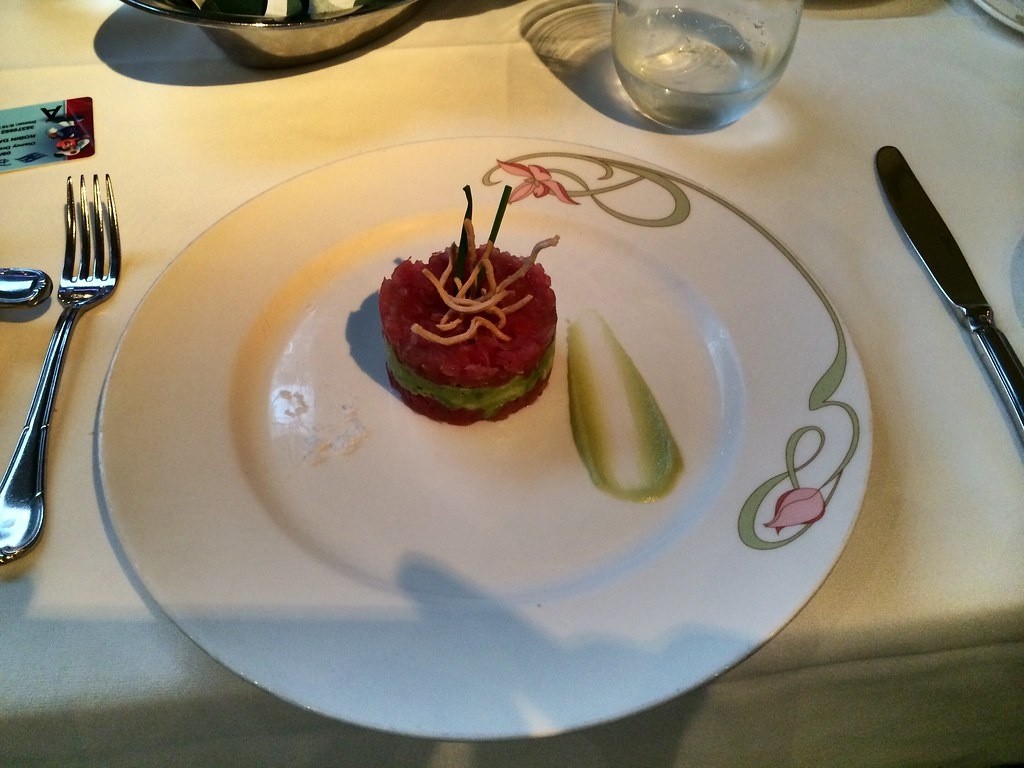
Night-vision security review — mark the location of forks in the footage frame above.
[0,176,122,566]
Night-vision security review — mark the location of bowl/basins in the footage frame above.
[121,0,421,69]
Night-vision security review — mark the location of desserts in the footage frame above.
[380,183,562,427]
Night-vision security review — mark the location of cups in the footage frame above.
[612,1,803,133]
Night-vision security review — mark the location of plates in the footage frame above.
[95,136,872,739]
[973,0,1024,34]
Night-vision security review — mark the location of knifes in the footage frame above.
[874,146,1024,448]
